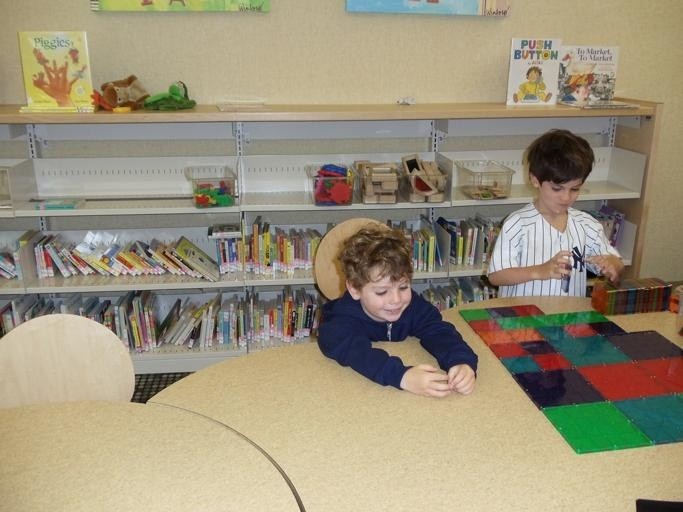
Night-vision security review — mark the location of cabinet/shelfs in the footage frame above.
[1,105,249,375]
[242,98,665,356]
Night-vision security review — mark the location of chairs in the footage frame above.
[0,314,136,402]
[312,218,395,300]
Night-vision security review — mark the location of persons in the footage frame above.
[512,67,552,102]
[316,228,479,399]
[485,130,626,297]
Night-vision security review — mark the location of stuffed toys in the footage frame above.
[194,181,234,209]
[91,75,197,112]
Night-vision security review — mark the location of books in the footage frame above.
[556,46,620,104]
[557,99,640,110]
[581,205,626,249]
[207,215,323,275]
[16,30,96,115]
[0,229,219,282]
[19,198,85,210]
[506,37,562,107]
[0,285,327,353]
[386,213,502,314]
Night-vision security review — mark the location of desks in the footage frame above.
[144,296,682,510]
[0,402,303,511]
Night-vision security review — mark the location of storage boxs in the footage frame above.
[451,157,516,203]
[303,162,356,208]
[181,163,238,209]
[356,158,403,207]
[394,160,449,205]
